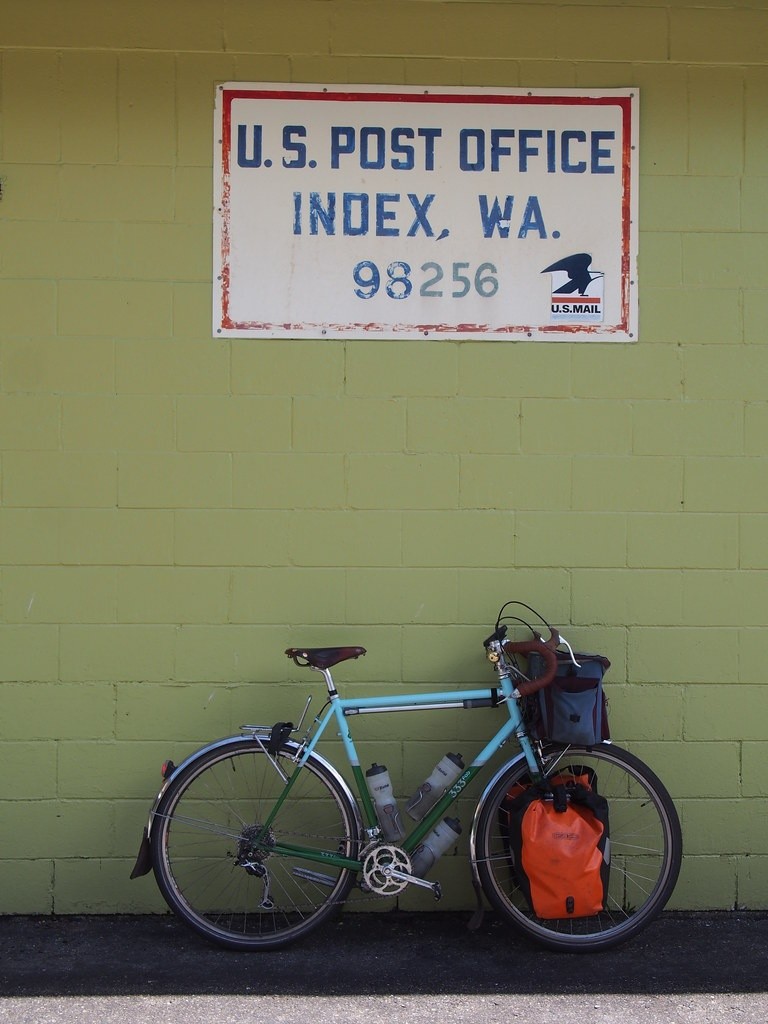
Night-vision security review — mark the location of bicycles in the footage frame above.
[127,602,684,956]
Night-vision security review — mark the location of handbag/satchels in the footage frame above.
[497,765,610,920]
[526,649,610,746]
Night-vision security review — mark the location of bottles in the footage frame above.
[407,752,470,821]
[391,817,461,883]
[365,761,406,842]
